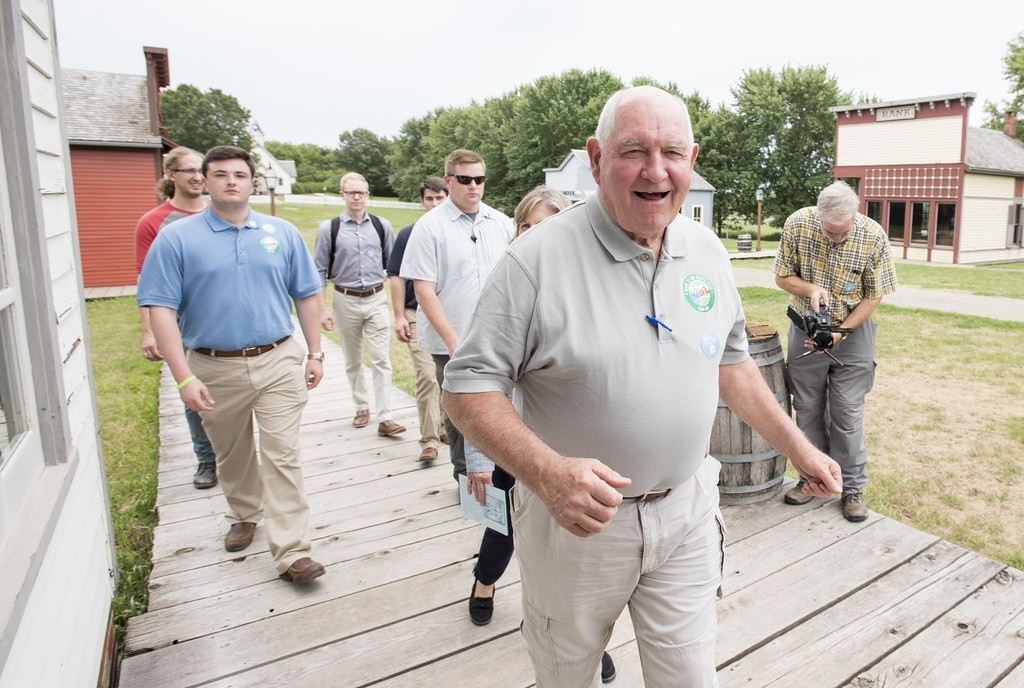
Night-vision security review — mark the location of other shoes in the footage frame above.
[469,578,496,626]
[601,651,616,684]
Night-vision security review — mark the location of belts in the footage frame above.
[194,336,291,360]
[334,284,384,298]
[622,489,670,504]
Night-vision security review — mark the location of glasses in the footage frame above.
[820,218,855,238]
[172,169,204,178]
[446,172,486,185]
[343,191,369,198]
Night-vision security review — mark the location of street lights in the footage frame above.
[755,189,764,251]
[264,163,277,215]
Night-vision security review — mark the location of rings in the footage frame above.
[144,353,148,357]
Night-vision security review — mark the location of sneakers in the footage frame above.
[842,493,868,521]
[784,478,815,505]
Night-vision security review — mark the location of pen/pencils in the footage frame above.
[646,315,673,333]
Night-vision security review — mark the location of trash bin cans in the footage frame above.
[711,321,791,505]
[737,234,753,253]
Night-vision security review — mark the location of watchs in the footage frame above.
[307,352,324,362]
[837,327,847,341]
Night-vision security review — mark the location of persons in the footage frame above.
[137,145,325,583]
[464,189,616,682]
[135,146,219,489]
[388,176,450,462]
[398,150,518,505]
[439,86,842,688]
[313,172,406,435]
[772,180,896,522]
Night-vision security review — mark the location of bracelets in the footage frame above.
[177,375,196,391]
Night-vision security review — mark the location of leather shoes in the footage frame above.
[353,409,369,427]
[419,447,438,462]
[378,420,406,436]
[225,521,257,552]
[440,434,450,445]
[279,558,326,585]
[194,462,217,488]
